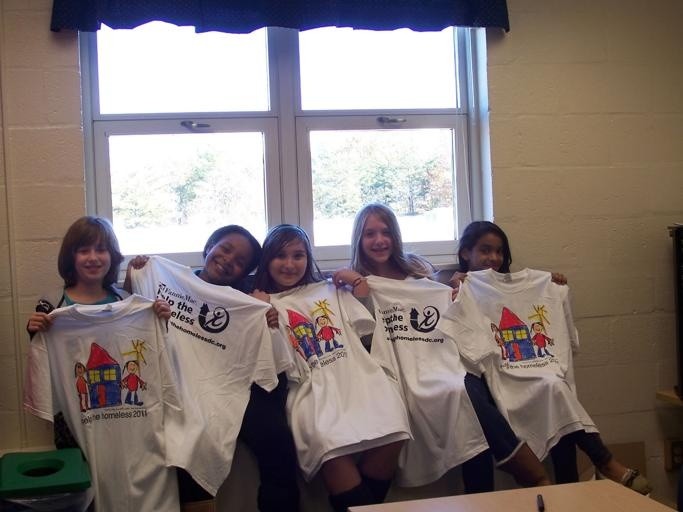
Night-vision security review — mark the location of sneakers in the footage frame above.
[595,463,653,495]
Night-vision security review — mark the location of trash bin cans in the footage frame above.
[0,448,94,512]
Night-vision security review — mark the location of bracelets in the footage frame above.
[349,274,368,289]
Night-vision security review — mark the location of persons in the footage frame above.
[254,224,407,512]
[24,214,171,512]
[450,221,653,497]
[348,205,555,502]
[123,221,281,512]
